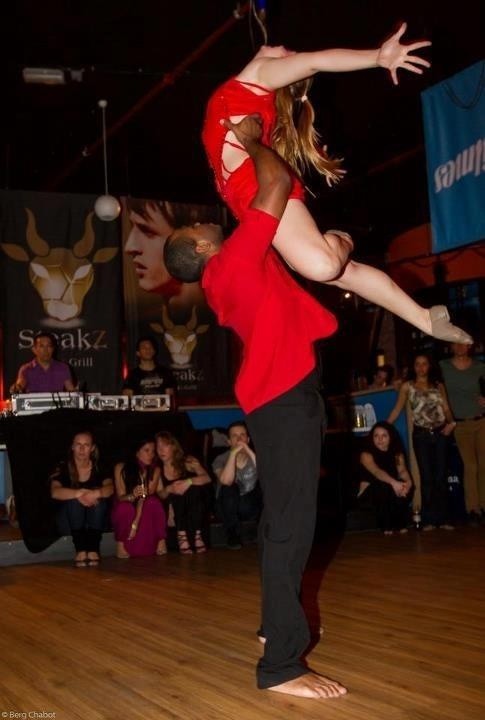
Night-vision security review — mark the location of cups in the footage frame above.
[355,415,365,427]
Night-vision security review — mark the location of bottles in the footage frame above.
[137,474,148,499]
[375,350,388,387]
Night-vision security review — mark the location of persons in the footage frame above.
[6,332,77,397]
[165,110,352,701]
[200,18,478,348]
[347,334,484,538]
[50,415,260,573]
[122,335,174,396]
[123,196,223,393]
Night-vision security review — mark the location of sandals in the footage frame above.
[74,534,242,568]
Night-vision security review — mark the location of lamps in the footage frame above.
[93,99,121,220]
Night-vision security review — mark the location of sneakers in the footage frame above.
[430,306,473,345]
